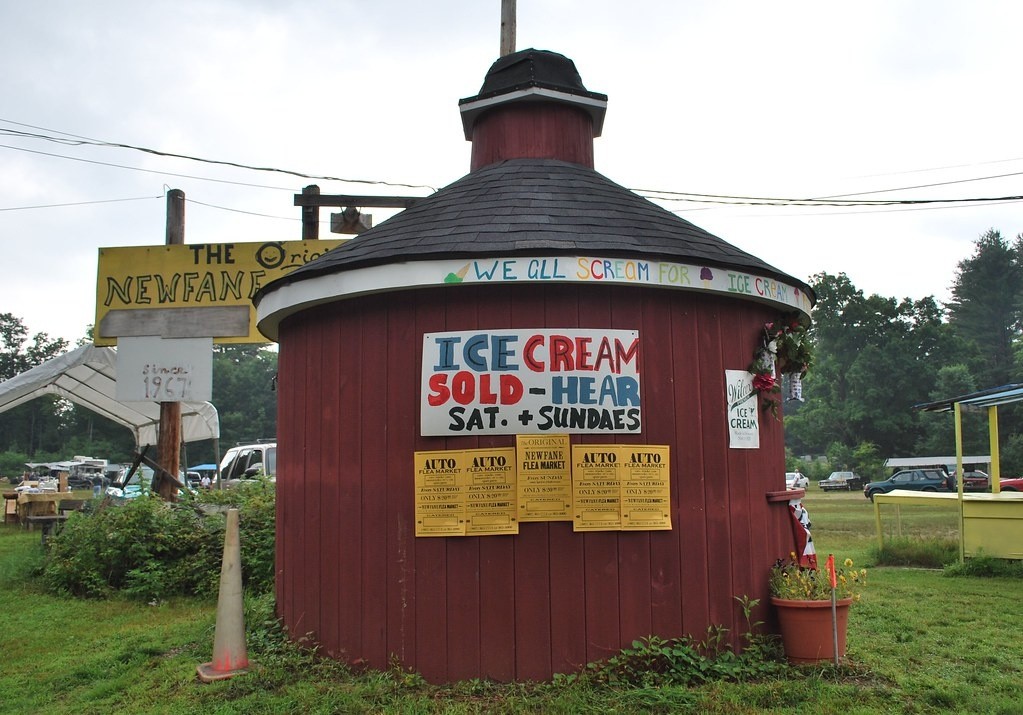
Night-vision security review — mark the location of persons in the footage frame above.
[92,472,102,499]
[201,474,211,485]
[793,469,800,487]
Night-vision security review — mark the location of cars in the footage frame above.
[819,469,865,491]
[103,463,202,488]
[950,469,1023,492]
[784,471,810,493]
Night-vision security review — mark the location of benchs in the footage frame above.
[27,515,70,547]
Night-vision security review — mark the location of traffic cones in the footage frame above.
[195,508,268,682]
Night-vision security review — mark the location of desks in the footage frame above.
[3,491,18,525]
[17,491,73,531]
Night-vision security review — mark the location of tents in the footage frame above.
[0,342,222,492]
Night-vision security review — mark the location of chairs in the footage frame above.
[58,499,84,517]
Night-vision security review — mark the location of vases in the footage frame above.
[771,595,855,664]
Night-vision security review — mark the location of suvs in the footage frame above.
[211,438,277,484]
[864,468,956,504]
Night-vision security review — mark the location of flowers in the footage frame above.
[769,549,868,603]
[730,317,816,420]
